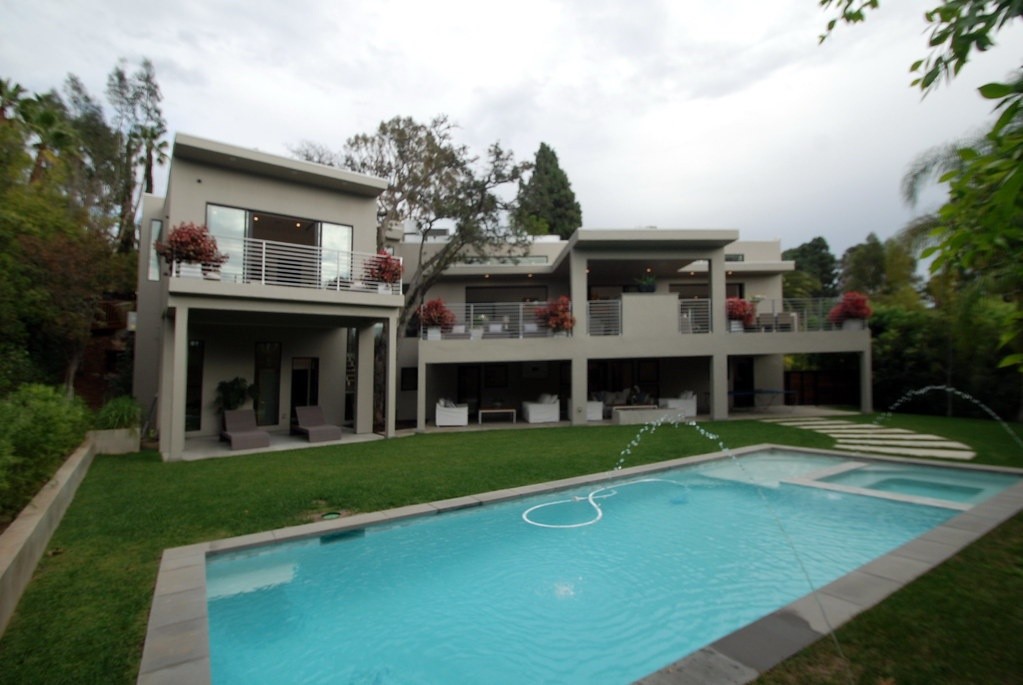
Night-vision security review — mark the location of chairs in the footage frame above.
[292,406,340,442]
[221,409,270,450]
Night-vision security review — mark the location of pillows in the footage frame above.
[445,400,456,407]
[681,390,693,399]
[439,398,445,407]
[539,394,551,403]
[549,395,558,403]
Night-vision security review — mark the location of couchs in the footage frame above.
[435,404,469,427]
[567,399,603,420]
[522,398,560,423]
[659,394,697,416]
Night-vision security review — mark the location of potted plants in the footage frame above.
[726,298,755,332]
[362,250,403,293]
[539,295,576,336]
[151,223,228,278]
[830,292,872,330]
[416,298,455,340]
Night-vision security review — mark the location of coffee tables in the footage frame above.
[605,404,658,418]
[478,408,517,424]
